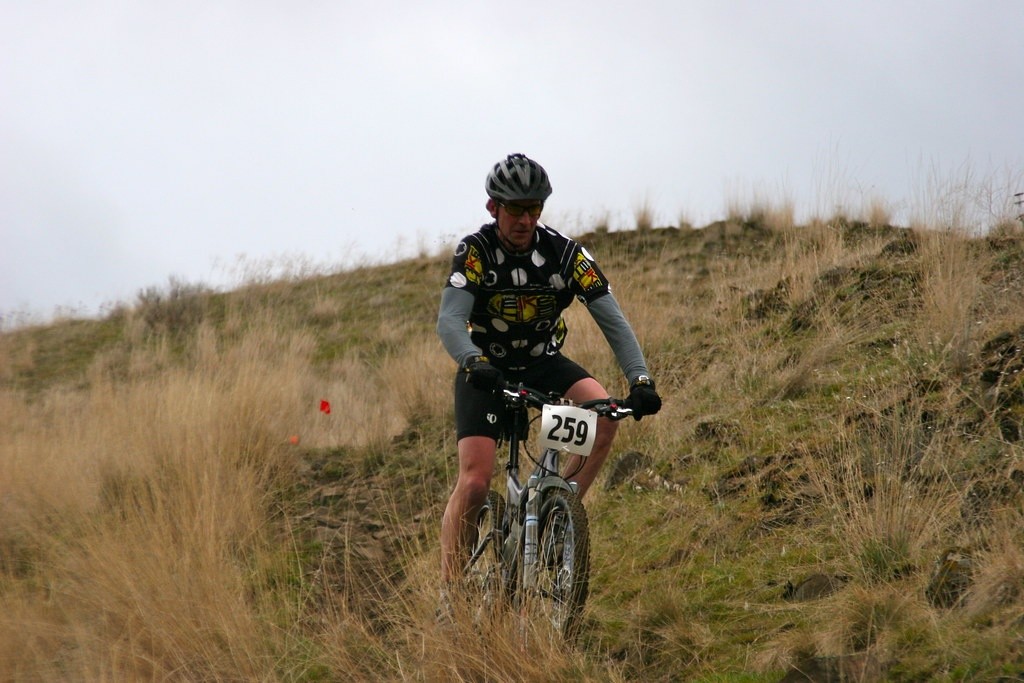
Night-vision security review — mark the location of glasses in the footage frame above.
[497,201,543,218]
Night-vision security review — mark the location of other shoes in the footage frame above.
[433,590,458,629]
[543,511,568,568]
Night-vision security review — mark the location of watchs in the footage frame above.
[631,375,648,386]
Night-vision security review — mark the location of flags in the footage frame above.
[320,400,331,415]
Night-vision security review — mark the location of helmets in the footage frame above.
[485,154,552,204]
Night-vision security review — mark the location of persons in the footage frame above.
[436,154,662,605]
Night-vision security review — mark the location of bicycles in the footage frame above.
[461,367,633,646]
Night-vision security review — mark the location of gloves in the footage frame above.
[624,379,663,422]
[464,355,500,387]
